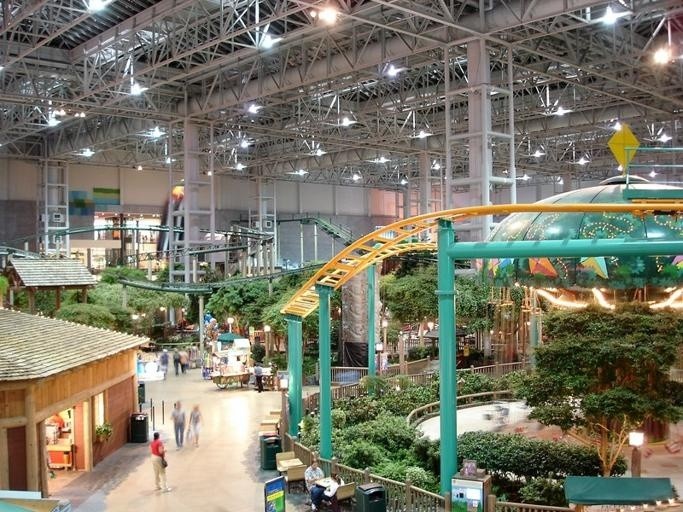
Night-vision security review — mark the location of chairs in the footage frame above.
[259,409,355,512]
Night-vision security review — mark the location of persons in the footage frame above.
[185,403,205,448]
[173,348,180,377]
[151,432,174,493]
[304,458,325,512]
[159,348,172,380]
[177,349,189,374]
[321,464,346,505]
[254,363,265,392]
[169,400,185,451]
[189,342,198,360]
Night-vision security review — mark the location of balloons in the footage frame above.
[202,309,218,345]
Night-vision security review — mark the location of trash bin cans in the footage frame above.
[131,414,148,442]
[138,384,145,403]
[463,345,469,357]
[355,483,386,512]
[260,435,281,470]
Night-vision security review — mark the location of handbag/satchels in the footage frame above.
[162,460,168,467]
